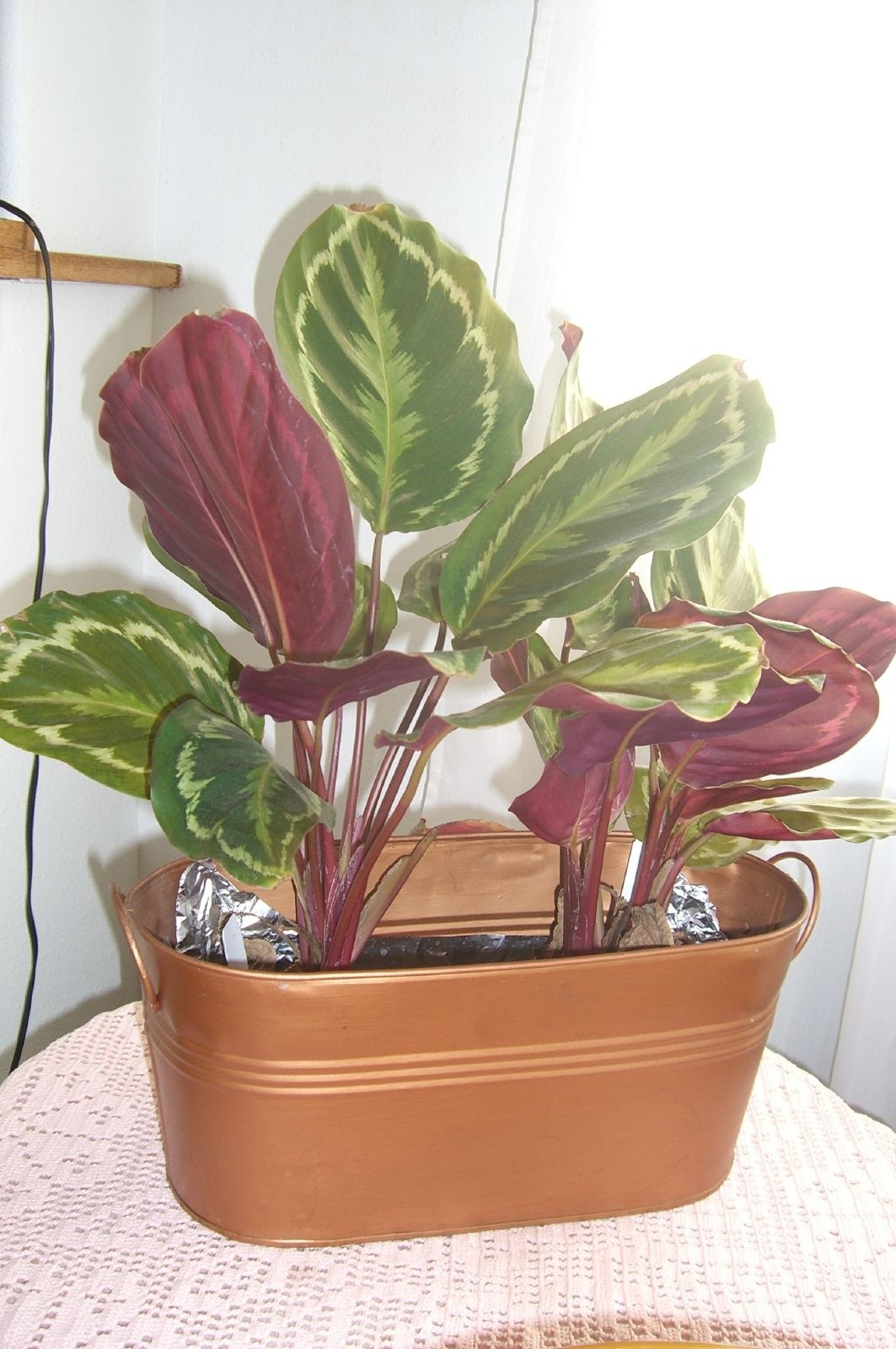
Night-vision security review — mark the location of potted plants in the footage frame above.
[0,199,896,1252]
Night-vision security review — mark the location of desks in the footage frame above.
[0,996,896,1349]
